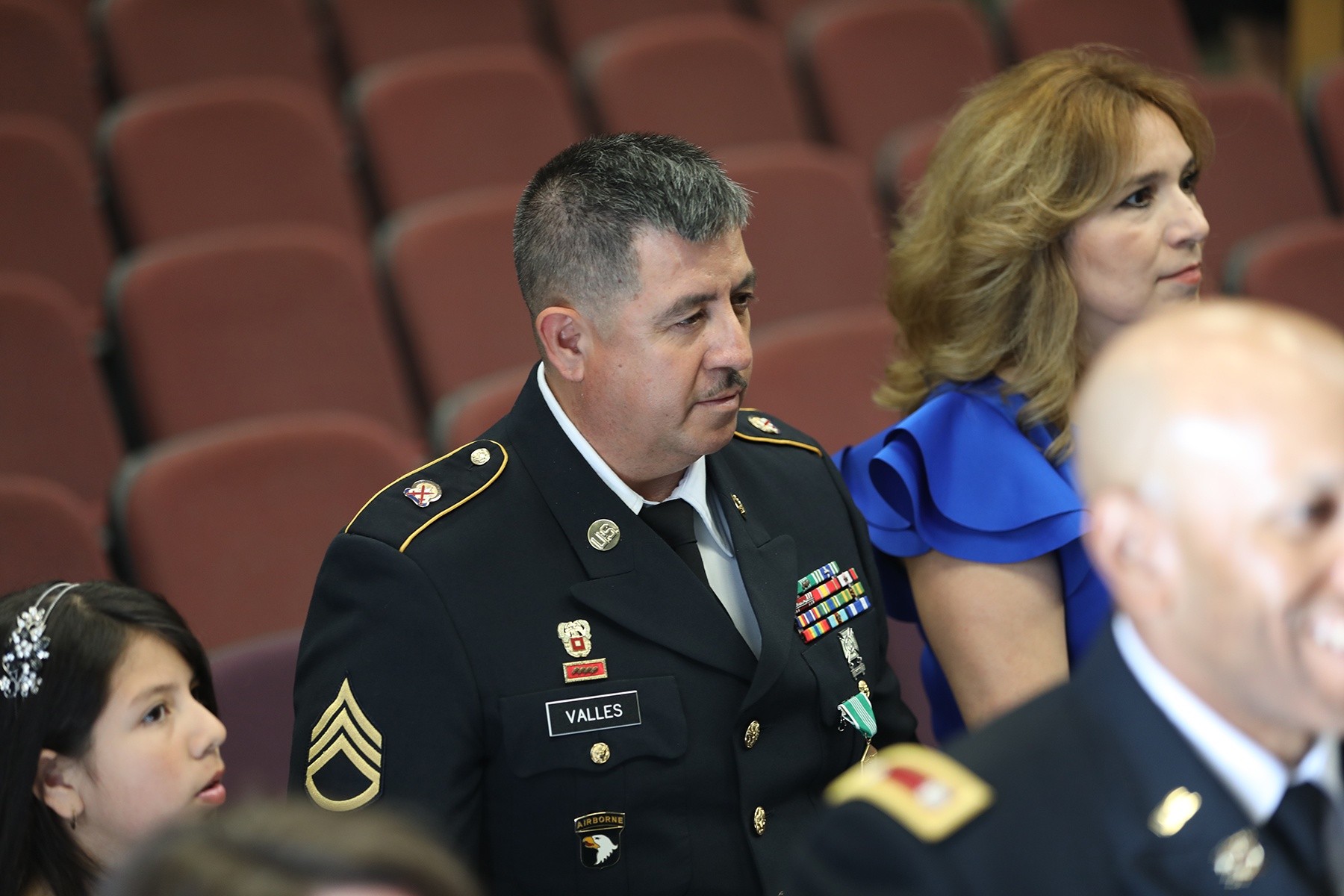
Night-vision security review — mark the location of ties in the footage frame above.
[1263,782,1335,896]
[637,498,736,627]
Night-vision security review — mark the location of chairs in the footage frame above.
[0,0,1344,816]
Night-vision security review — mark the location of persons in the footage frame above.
[285,127,921,896]
[774,302,1344,896]
[0,580,230,896]
[816,39,1210,741]
